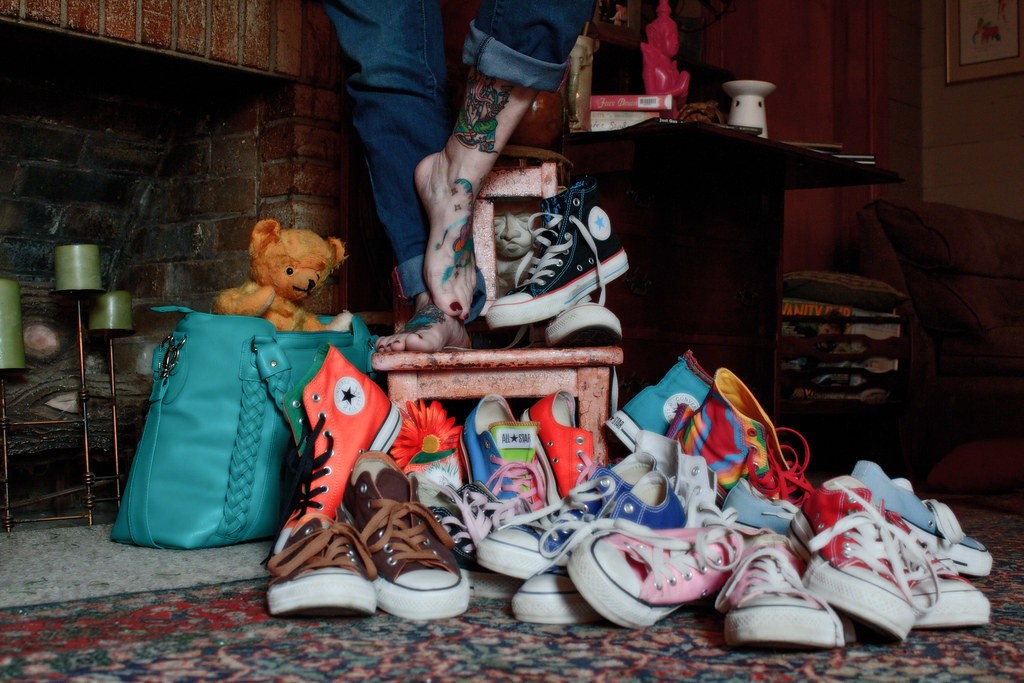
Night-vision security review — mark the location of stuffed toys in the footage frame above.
[211,219,353,334]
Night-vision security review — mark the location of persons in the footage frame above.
[321,0,597,352]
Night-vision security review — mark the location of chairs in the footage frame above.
[378,159,636,464]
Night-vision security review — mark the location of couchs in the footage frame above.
[858,192,1024,497]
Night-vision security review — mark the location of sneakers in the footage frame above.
[487,173,631,329]
[567,521,747,629]
[632,430,738,528]
[718,477,798,539]
[475,451,659,581]
[343,449,470,621]
[521,387,596,506]
[714,529,846,646]
[603,350,714,452]
[784,474,918,640]
[267,507,377,614]
[459,392,550,516]
[673,367,816,511]
[511,470,686,626]
[544,295,624,346]
[848,460,994,578]
[408,471,526,600]
[882,507,990,628]
[272,342,403,557]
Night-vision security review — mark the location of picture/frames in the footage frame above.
[945,1,1024,88]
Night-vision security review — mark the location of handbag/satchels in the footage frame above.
[111,310,377,549]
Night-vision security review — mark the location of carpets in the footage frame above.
[0,507,1024,683]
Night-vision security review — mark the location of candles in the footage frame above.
[53,244,104,289]
[0,276,28,369]
[89,291,131,331]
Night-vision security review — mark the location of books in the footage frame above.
[780,297,902,401]
[591,94,672,133]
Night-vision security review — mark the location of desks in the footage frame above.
[568,121,904,416]
